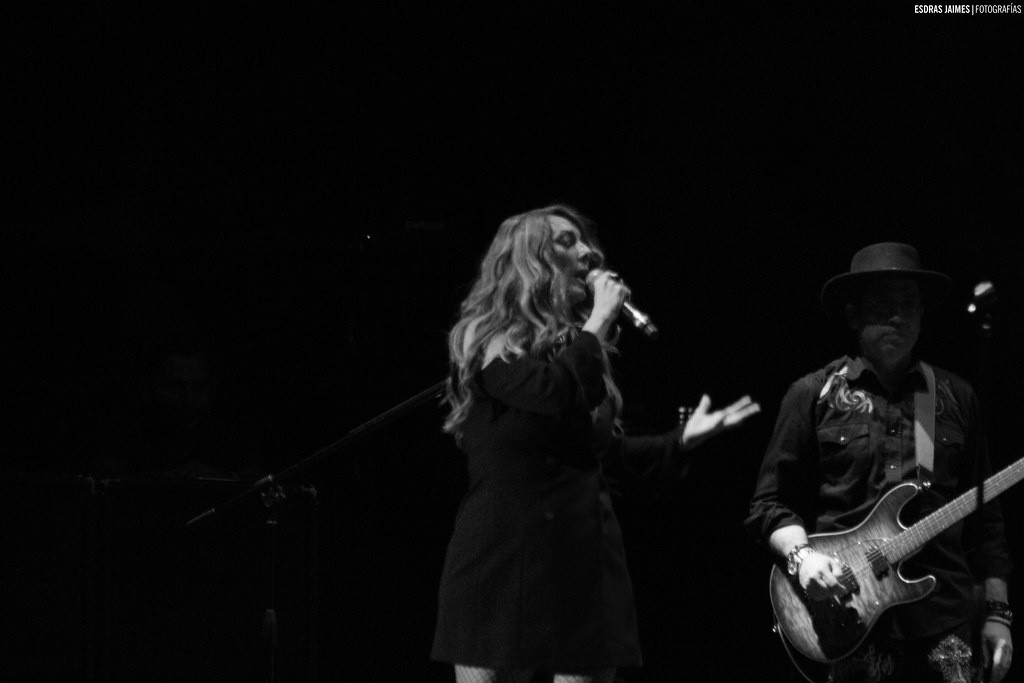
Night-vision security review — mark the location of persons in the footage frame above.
[430,204,760,683]
[738,242,1014,683]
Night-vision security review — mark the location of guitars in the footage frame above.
[769,452,1024,665]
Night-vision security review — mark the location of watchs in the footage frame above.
[786,548,816,577]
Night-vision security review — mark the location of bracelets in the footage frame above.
[985,599,1013,630]
[783,543,812,562]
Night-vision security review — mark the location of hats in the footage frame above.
[819,241,949,316]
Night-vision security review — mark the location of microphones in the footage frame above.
[586,268,658,335]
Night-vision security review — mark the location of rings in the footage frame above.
[608,273,620,282]
